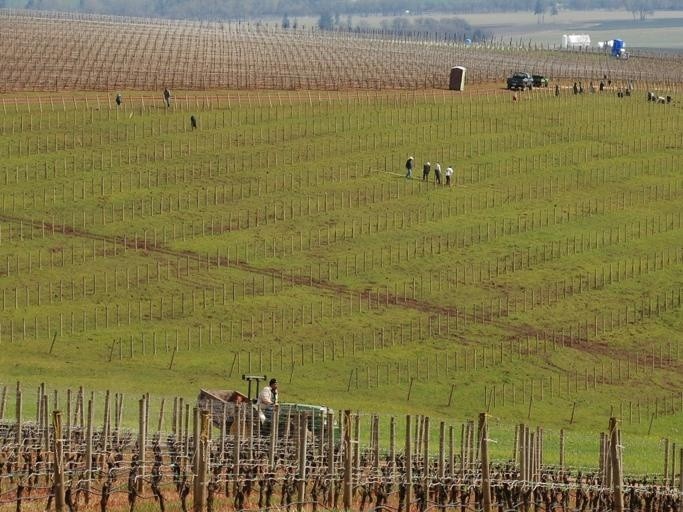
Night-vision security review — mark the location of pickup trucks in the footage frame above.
[506,71,535,91]
[532,75,550,87]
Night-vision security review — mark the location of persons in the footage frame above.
[114,93,122,111]
[190,115,196,131]
[444,165,453,185]
[163,86,172,108]
[404,155,414,177]
[435,161,442,184]
[256,378,279,418]
[556,72,673,106]
[421,161,432,182]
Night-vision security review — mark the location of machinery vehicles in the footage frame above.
[194,373,340,438]
